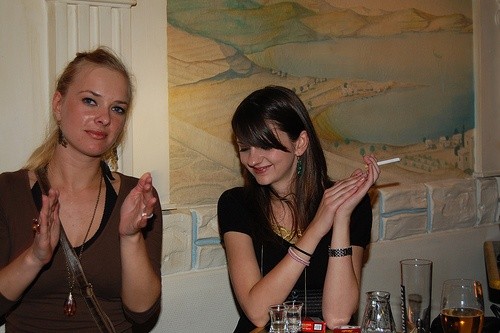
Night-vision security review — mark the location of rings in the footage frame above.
[31,218,40,230]
[143,211,153,218]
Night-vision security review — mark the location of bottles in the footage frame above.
[360,290,398,333]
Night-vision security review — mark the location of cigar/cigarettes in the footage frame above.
[365,156,401,168]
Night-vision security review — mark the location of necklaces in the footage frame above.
[272,225,303,242]
[63,173,102,316]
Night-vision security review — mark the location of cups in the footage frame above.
[282,300,304,333]
[440,278,485,333]
[268,304,290,333]
[400,257,433,333]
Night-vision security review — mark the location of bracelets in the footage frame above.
[288,243,312,267]
[328,246,352,257]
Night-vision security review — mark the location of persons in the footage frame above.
[0,49,163,333]
[217,84,381,333]
